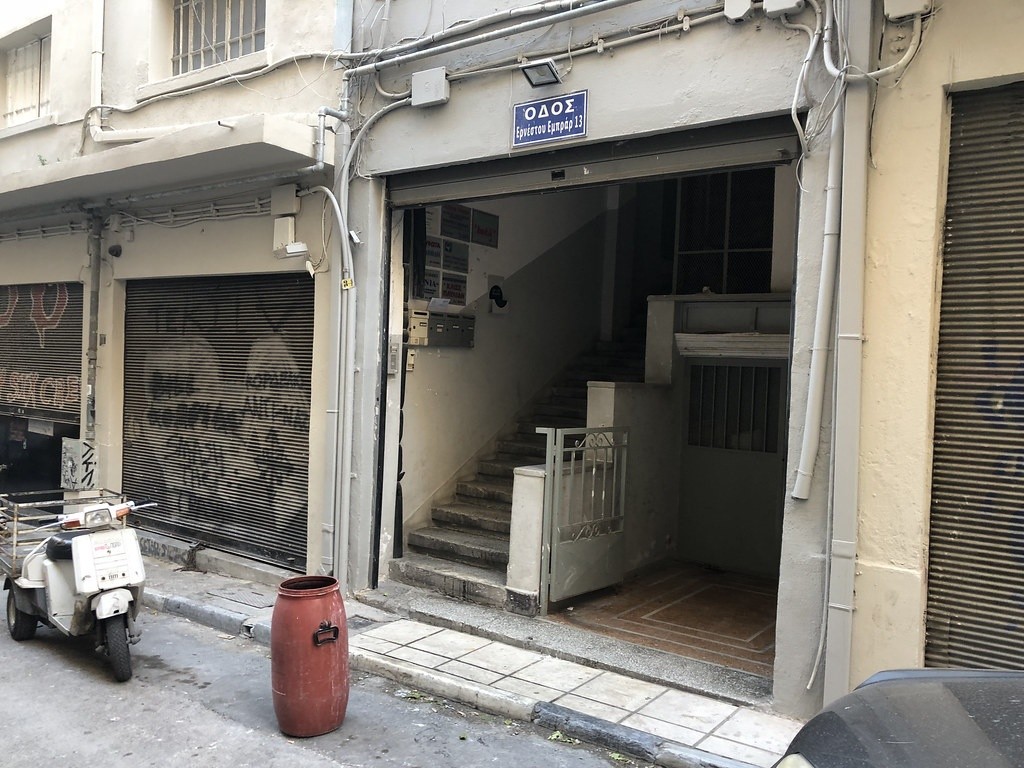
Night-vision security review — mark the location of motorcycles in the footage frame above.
[3,499,158,681]
